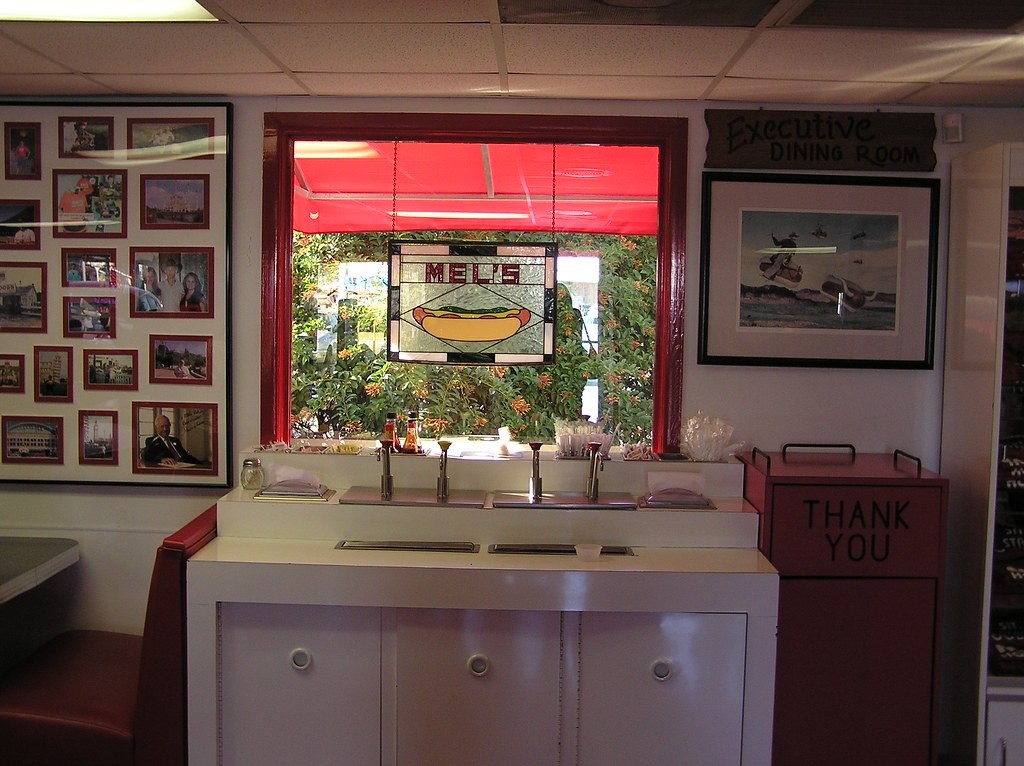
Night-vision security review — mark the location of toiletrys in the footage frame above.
[402,411,423,454]
[382,412,401,453]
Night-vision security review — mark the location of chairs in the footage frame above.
[0,504,216,766]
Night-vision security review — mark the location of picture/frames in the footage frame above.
[148,333,212,386]
[52,169,128,239]
[33,345,73,403]
[61,247,117,289]
[139,173,212,230]
[57,116,116,159]
[82,348,139,391]
[0,354,25,394]
[0,261,49,334]
[699,172,941,370]
[131,399,218,475]
[129,246,215,318]
[77,409,119,466]
[0,199,41,251]
[126,117,216,161]
[2,415,66,464]
[62,296,118,339]
[4,121,42,181]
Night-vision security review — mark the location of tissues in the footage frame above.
[252,462,337,502]
[637,471,718,510]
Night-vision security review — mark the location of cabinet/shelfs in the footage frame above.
[739,452,950,766]
[931,143,1024,766]
[185,538,777,765]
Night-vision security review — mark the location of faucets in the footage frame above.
[437,440,452,497]
[376,440,394,498]
[585,442,604,500]
[528,442,543,500]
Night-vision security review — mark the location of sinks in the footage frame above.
[487,543,635,556]
[334,539,481,553]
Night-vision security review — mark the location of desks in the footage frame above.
[0,536,81,606]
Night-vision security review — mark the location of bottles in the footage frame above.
[384,411,422,454]
[241,458,263,491]
